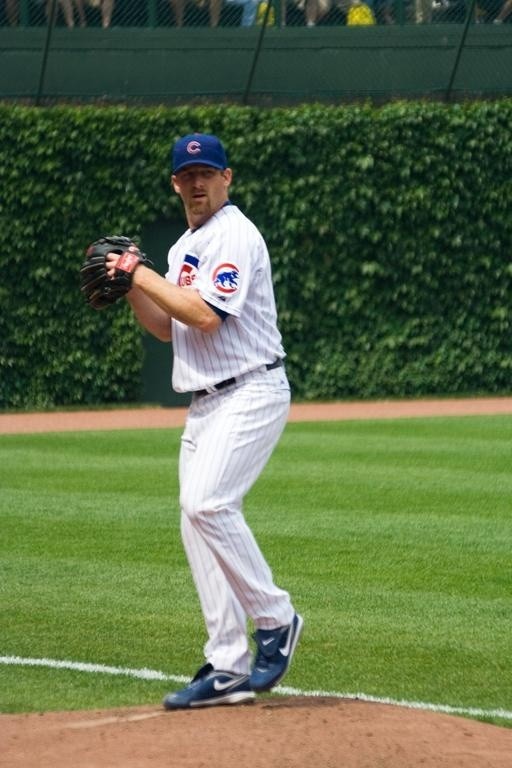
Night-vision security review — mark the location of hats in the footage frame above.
[170,133,229,176]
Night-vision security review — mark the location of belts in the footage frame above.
[195,357,284,397]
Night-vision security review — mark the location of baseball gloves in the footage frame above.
[79,235,153,310]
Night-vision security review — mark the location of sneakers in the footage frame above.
[162,661,258,710]
[249,613,303,694]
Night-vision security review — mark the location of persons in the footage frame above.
[0,1,512,35]
[78,133,304,710]
